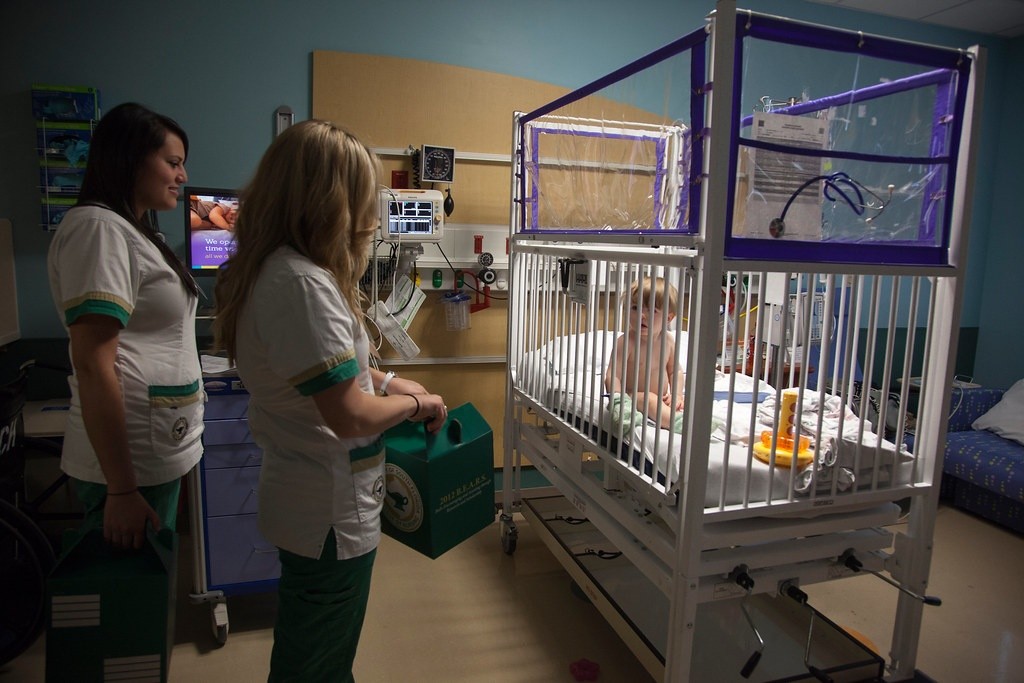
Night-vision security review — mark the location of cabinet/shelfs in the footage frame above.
[189,372,285,644]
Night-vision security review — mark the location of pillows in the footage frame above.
[971,377,1024,445]
[540,330,719,374]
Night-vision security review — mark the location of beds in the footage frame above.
[500,328,942,683]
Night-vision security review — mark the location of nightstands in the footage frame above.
[716,362,814,391]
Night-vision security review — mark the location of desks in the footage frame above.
[23,399,70,437]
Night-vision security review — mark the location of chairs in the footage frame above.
[797,287,915,453]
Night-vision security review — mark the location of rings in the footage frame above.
[445,405,447,411]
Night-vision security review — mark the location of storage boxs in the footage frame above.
[42,512,179,683]
[379,401,495,560]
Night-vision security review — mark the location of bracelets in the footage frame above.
[108,489,138,495]
[379,370,398,396]
[403,392,420,418]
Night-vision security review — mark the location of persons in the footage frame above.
[190,194,238,231]
[48,100,208,546]
[605,276,719,437]
[216,116,448,683]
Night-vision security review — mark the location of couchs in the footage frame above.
[939,387,1024,534]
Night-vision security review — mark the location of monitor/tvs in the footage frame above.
[183,186,240,277]
[379,188,444,240]
